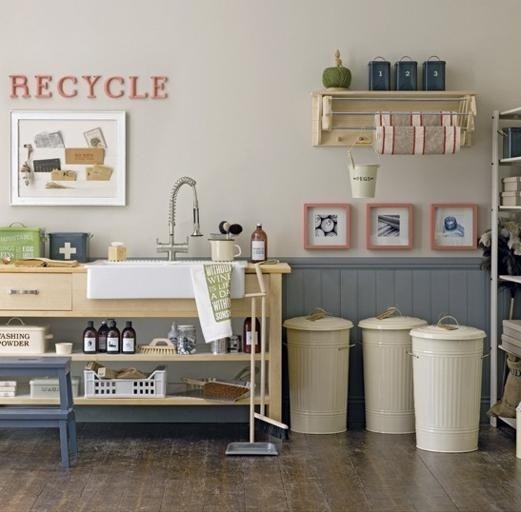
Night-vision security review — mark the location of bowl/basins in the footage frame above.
[55,342,73,354]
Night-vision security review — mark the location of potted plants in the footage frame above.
[322,50,352,88]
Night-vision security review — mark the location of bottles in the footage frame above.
[177,324,196,355]
[249,224,268,262]
[208,335,242,355]
[166,323,177,347]
[83,318,137,353]
[108,242,128,262]
[244,316,261,353]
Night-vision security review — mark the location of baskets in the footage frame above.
[84,371,166,399]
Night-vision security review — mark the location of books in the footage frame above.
[0,381,17,397]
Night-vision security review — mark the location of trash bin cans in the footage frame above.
[409,315,489,454]
[357,307,428,434]
[283,307,356,435]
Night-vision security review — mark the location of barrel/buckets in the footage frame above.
[347,142,380,200]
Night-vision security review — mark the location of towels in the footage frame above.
[371,109,459,129]
[374,125,459,155]
[189,256,232,342]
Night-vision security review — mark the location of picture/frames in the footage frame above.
[364,202,413,250]
[431,201,478,251]
[303,202,351,250]
[8,111,127,208]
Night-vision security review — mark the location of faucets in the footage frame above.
[154,176,204,261]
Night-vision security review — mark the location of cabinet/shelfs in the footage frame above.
[488,105,521,431]
[310,88,476,149]
[0,261,292,436]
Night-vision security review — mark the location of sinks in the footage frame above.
[83,259,248,302]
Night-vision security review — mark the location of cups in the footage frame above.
[208,238,242,262]
[346,163,379,200]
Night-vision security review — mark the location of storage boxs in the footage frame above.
[0,318,54,355]
[29,377,80,398]
[47,231,90,263]
